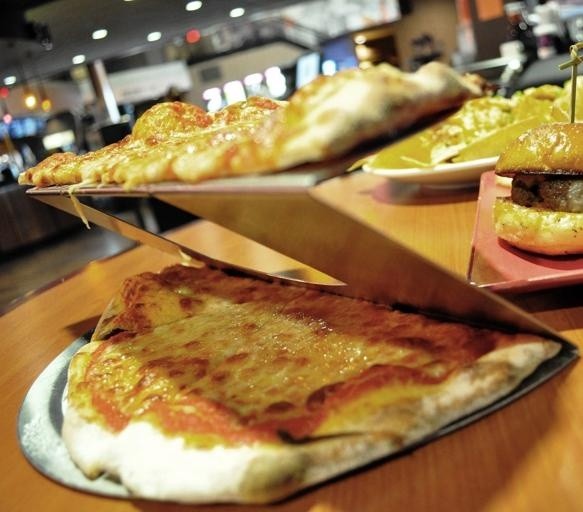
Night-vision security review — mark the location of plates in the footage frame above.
[465,168,582,296]
[359,153,500,192]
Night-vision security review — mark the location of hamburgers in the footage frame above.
[492,120,582,256]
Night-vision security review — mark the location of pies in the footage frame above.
[345,77,583,173]
[60,261,564,507]
[16,60,483,186]
